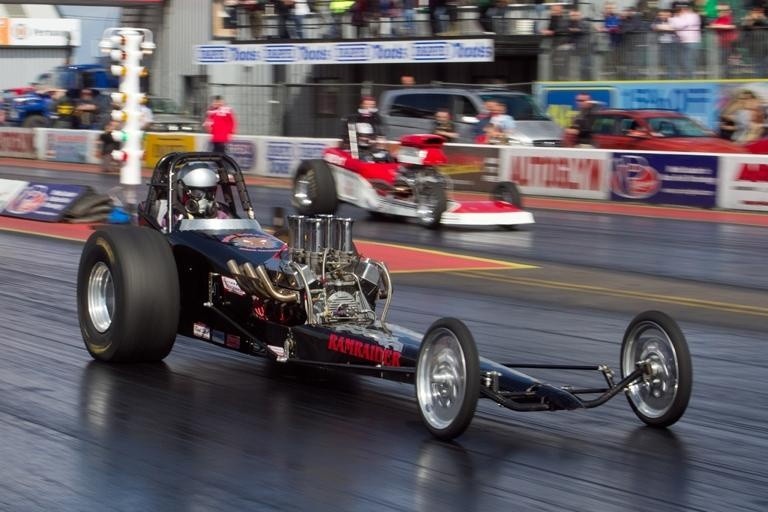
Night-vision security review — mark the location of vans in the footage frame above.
[378,88,563,146]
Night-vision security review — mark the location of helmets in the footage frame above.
[177,162,219,215]
[354,123,374,148]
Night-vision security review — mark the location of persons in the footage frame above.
[222,0,500,39]
[564,92,604,147]
[157,162,232,231]
[99,122,120,175]
[719,89,757,142]
[706,3,742,49]
[536,4,565,38]
[592,3,622,33]
[742,5,768,57]
[69,87,99,129]
[720,98,768,142]
[563,9,589,35]
[650,1,703,44]
[648,9,680,34]
[474,100,518,145]
[434,110,454,141]
[342,96,382,153]
[203,95,236,156]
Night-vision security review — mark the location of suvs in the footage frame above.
[1,66,109,128]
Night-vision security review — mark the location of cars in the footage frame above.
[561,108,746,154]
[146,96,202,132]
